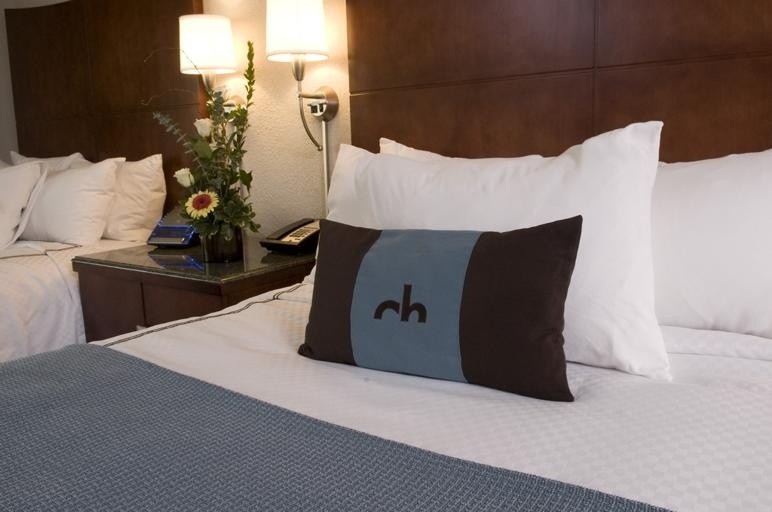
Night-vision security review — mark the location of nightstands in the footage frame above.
[70,233,315,344]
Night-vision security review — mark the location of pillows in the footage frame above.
[650,148,772,339]
[0,150,167,250]
[327,121,664,377]
[297,214,584,403]
[379,134,450,162]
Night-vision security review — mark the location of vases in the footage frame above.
[198,226,242,263]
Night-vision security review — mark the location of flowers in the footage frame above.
[151,41,260,239]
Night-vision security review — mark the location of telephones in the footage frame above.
[259,218,320,253]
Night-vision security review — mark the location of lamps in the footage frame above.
[264,0,339,216]
[178,13,239,124]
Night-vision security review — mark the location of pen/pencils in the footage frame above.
[184,254,204,271]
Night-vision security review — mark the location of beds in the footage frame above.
[0,147,772,510]
[0,239,164,364]
